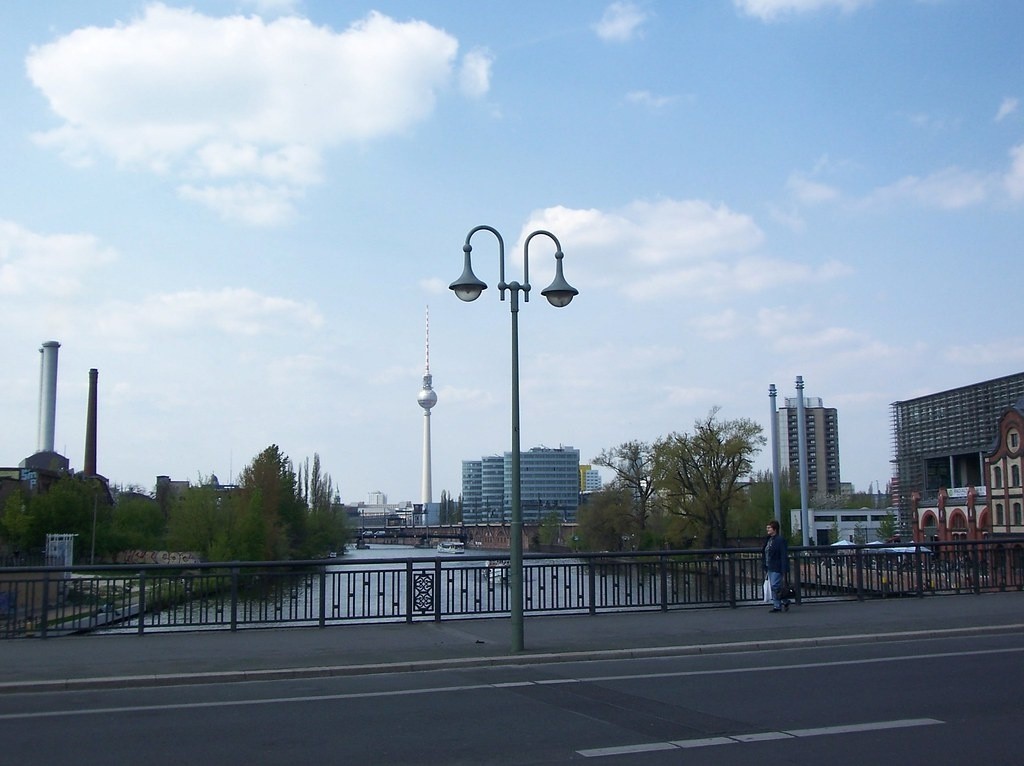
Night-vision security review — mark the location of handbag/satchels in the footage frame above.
[775,572,795,600]
[763,573,773,602]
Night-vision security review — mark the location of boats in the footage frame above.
[436,542,465,554]
[480,560,513,584]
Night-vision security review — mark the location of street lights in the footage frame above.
[449,224,580,652]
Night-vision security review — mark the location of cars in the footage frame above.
[363,530,385,539]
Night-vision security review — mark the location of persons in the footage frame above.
[761,519,792,615]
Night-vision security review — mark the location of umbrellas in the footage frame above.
[828,539,931,570]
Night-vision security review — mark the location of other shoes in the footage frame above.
[784,600,791,611]
[769,608,782,613]
